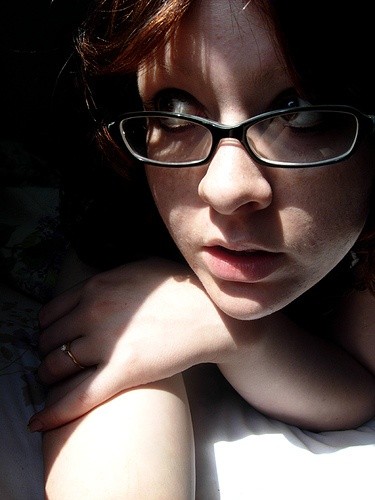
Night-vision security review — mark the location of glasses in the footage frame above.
[119,106,375,168]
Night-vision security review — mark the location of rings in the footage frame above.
[60,342,85,370]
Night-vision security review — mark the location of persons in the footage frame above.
[25,0,375,500]
